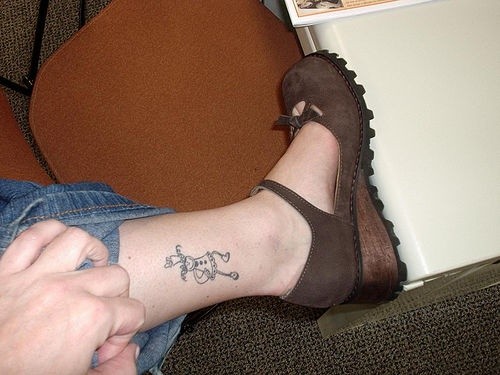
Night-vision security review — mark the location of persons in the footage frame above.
[0,47,408,375]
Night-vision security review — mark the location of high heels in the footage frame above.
[249,49,408,308]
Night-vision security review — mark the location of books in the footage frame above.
[284,1,432,28]
